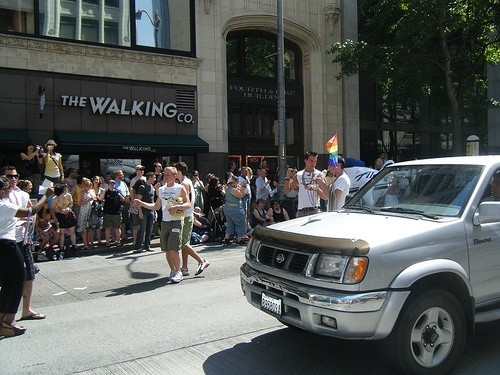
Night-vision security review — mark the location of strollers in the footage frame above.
[209,197,237,245]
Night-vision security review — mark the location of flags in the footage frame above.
[326,135,339,169]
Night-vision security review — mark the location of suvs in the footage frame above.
[240,154,500,375]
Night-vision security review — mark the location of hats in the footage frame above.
[45,140,58,147]
[135,165,145,170]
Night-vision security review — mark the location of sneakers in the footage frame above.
[171,271,183,284]
[169,272,176,281]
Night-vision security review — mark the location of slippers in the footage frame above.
[20,313,46,320]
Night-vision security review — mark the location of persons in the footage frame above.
[189,159,298,246]
[284,151,328,217]
[374,152,419,195]
[134,171,156,253]
[152,163,167,237]
[0,176,55,336]
[20,140,148,261]
[173,163,210,276]
[0,166,46,320]
[133,166,192,283]
[316,155,351,211]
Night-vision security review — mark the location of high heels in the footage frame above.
[0,320,26,337]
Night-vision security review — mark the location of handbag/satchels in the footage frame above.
[67,212,76,224]
[97,202,104,218]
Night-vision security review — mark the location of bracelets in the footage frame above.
[285,177,290,181]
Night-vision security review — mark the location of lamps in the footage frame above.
[136,9,160,31]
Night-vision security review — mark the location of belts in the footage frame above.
[298,207,321,212]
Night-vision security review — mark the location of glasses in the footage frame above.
[138,169,145,171]
[6,174,18,179]
[192,174,199,177]
[1,185,12,191]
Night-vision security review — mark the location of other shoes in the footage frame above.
[145,246,154,251]
[180,268,189,275]
[83,246,92,250]
[137,247,143,253]
[72,245,81,251]
[38,248,45,254]
[191,235,250,245]
[117,240,121,246]
[106,241,110,247]
[194,260,210,276]
[59,247,66,253]
[121,237,129,241]
[48,247,56,254]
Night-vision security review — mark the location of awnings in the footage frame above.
[0,128,209,156]
